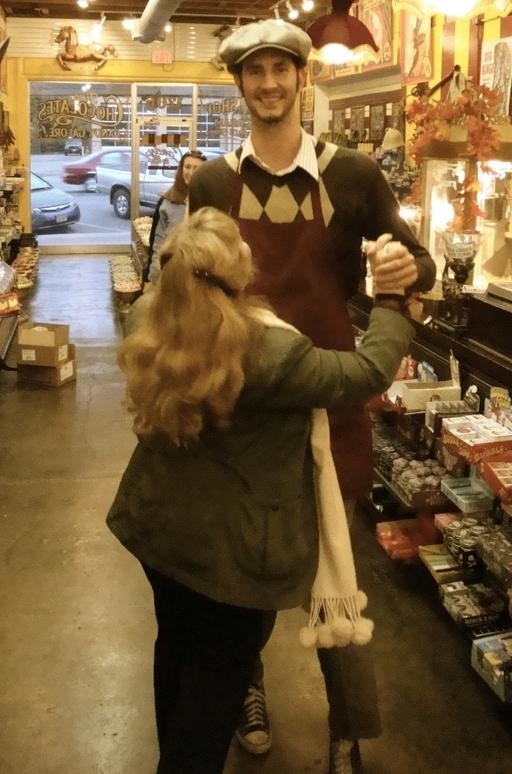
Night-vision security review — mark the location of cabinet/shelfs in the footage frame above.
[361,334,512,705]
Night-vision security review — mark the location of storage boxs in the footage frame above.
[19,345,77,388]
[16,319,70,366]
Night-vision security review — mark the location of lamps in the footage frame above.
[304,1,380,67]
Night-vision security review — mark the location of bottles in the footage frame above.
[443,516,488,569]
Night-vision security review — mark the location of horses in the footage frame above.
[55,26,117,70]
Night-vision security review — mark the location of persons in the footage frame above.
[180,14,439,774]
[131,148,211,293]
[104,203,418,774]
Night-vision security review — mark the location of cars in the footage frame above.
[31,169,81,233]
[62,145,229,192]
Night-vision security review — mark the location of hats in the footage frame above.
[219,17,309,68]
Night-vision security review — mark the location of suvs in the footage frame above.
[65,137,86,155]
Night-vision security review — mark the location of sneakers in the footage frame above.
[326,732,362,774]
[232,685,275,755]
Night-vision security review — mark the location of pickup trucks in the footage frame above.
[95,147,221,219]
